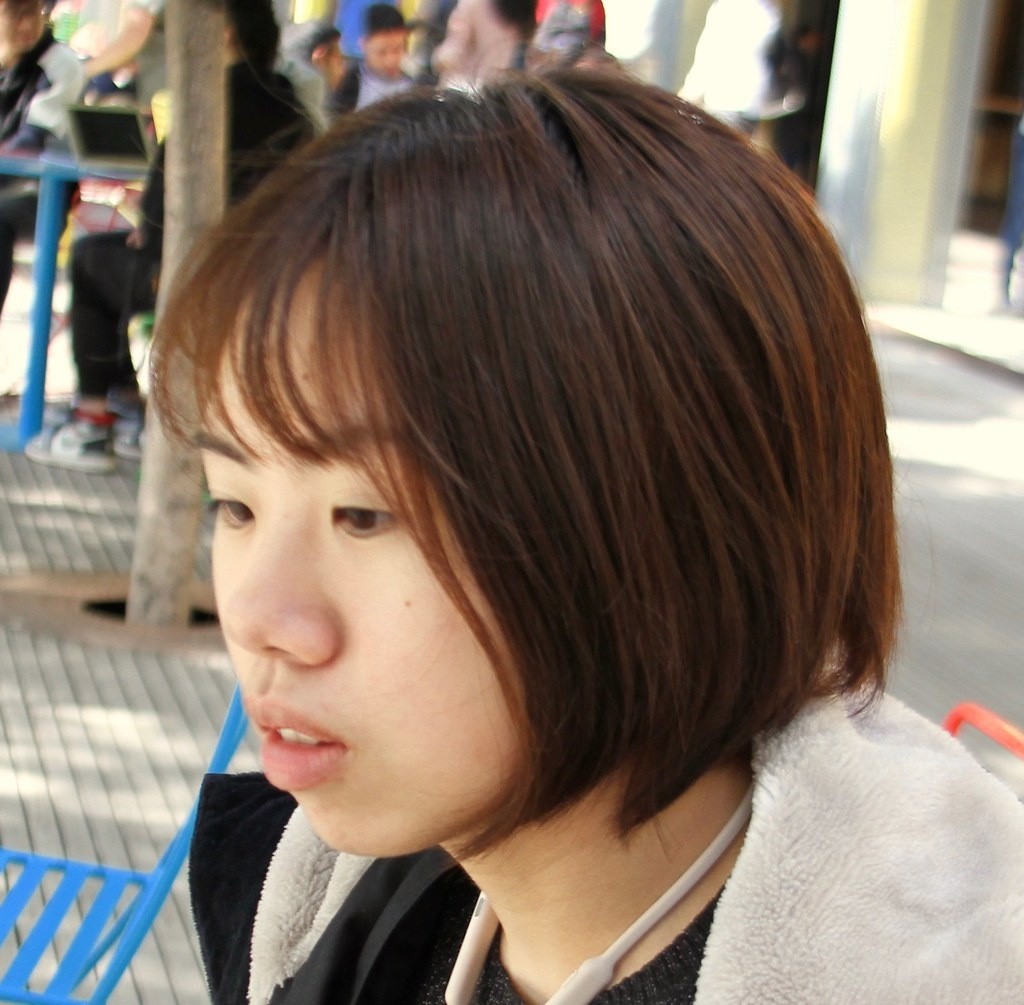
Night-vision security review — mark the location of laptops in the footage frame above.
[65,104,155,174]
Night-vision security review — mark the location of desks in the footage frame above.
[0,158,148,452]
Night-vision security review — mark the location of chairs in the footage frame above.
[0,686,245,1005]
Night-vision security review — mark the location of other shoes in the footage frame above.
[111,418,141,460]
[24,423,114,471]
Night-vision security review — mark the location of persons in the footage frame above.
[675,0,828,186]
[22,0,319,474]
[0,0,80,311]
[146,66,1024,1005]
[1000,112,1024,313]
[49,0,164,158]
[283,0,621,131]
